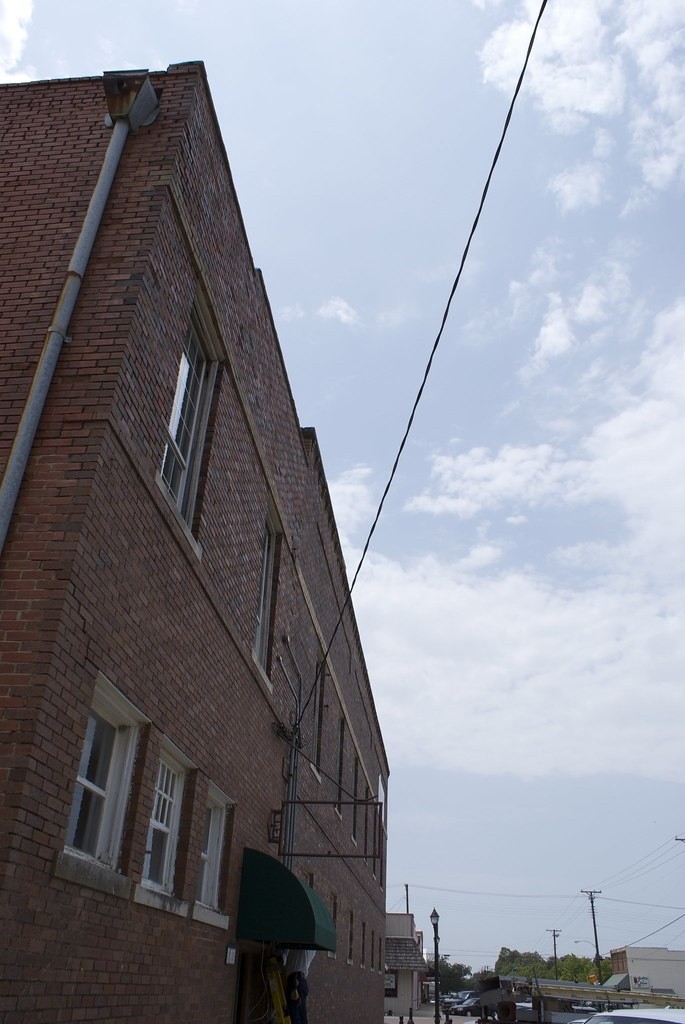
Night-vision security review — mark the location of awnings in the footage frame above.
[603,973,630,989]
[427,965,440,978]
[236,847,337,953]
[418,972,431,982]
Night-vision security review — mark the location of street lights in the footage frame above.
[574,940,603,984]
[429,907,441,1024]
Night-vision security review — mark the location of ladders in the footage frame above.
[265,956,293,1024]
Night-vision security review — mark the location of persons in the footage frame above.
[283,949,316,1024]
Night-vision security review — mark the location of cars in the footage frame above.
[450,998,482,1019]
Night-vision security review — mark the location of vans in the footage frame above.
[443,991,484,1011]
